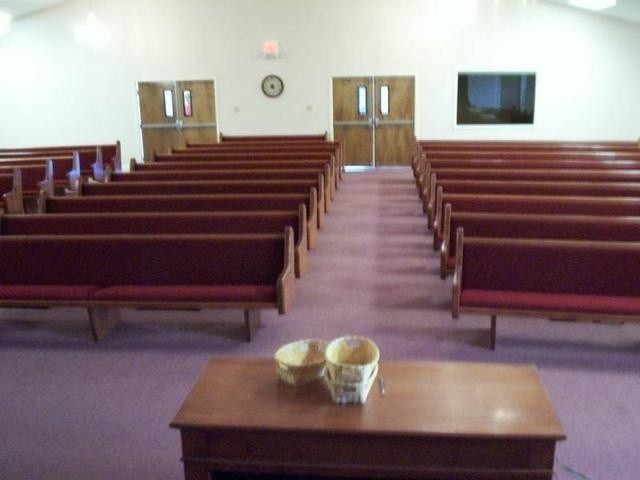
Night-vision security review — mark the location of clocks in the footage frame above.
[261,73,285,98]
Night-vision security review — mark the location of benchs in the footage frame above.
[0,131,344,342]
[409,139,640,351]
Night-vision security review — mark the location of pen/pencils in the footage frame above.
[379,376,385,395]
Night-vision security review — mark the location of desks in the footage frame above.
[166,354,569,480]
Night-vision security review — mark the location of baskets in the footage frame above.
[324,334,380,382]
[321,365,380,404]
[273,338,326,386]
